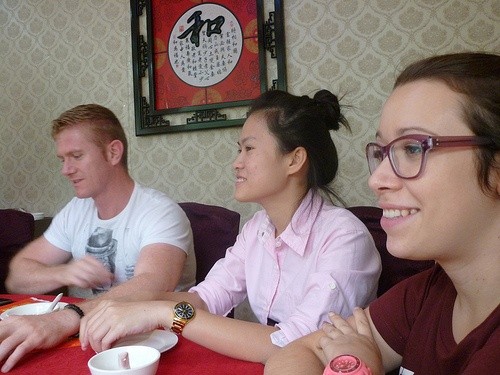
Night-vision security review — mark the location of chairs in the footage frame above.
[0,209,34,293]
[347,206,434,300]
[180,203,240,318]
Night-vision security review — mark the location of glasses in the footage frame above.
[366,134,495,179]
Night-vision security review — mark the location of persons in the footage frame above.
[79,90,381,364]
[0,104,196,375]
[263,53,500,375]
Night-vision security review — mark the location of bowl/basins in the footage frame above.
[6,305,61,317]
[88,346,160,375]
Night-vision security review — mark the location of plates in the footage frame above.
[113,329,179,353]
[0,301,69,320]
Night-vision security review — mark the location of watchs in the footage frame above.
[171,301,196,336]
[323,354,372,375]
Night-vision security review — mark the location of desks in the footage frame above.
[0,295,267,375]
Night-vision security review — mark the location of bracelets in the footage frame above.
[64,302,84,338]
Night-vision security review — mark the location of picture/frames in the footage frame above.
[130,0,287,137]
